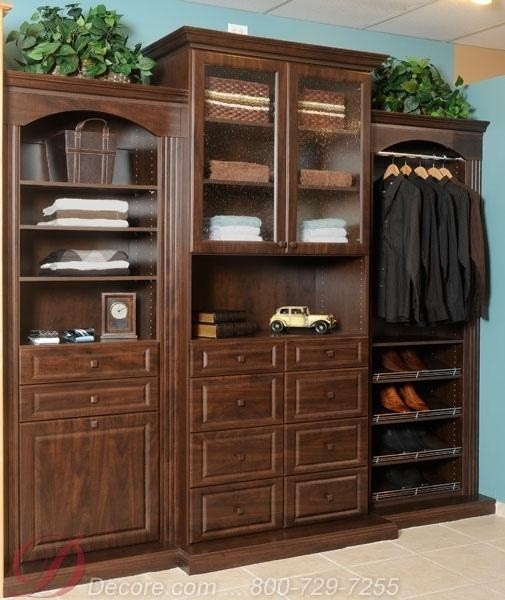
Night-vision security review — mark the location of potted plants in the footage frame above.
[4,3,157,84]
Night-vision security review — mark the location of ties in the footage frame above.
[63,326,96,343]
[28,329,60,344]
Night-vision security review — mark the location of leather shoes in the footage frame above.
[379,347,427,371]
[373,424,424,456]
[384,464,431,490]
[375,382,430,414]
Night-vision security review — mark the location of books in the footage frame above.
[194,324,258,338]
[198,311,247,325]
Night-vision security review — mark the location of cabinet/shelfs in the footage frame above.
[3,24,497,598]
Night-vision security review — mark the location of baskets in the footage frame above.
[51,57,131,82]
